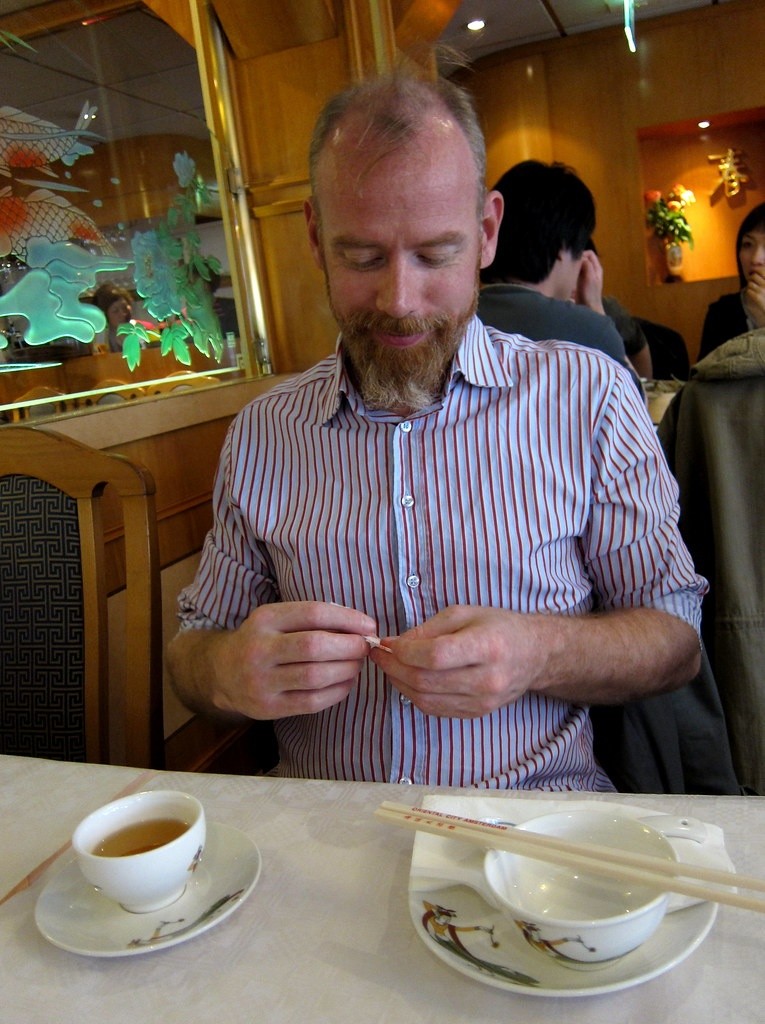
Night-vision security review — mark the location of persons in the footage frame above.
[85,257,240,355]
[475,159,653,383]
[696,202,764,362]
[163,62,711,790]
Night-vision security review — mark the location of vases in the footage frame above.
[657,237,685,283]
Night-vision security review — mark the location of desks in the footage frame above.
[0,754,764,1023]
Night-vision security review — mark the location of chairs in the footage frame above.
[0,425,164,769]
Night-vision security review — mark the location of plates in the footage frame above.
[407,885,718,996]
[35,823,261,958]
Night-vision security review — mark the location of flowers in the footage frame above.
[643,183,696,251]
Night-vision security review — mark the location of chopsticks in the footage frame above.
[373,802,765,911]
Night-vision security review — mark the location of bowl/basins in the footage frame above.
[71,790,205,913]
[484,809,678,962]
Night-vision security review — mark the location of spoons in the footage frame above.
[476,815,706,852]
